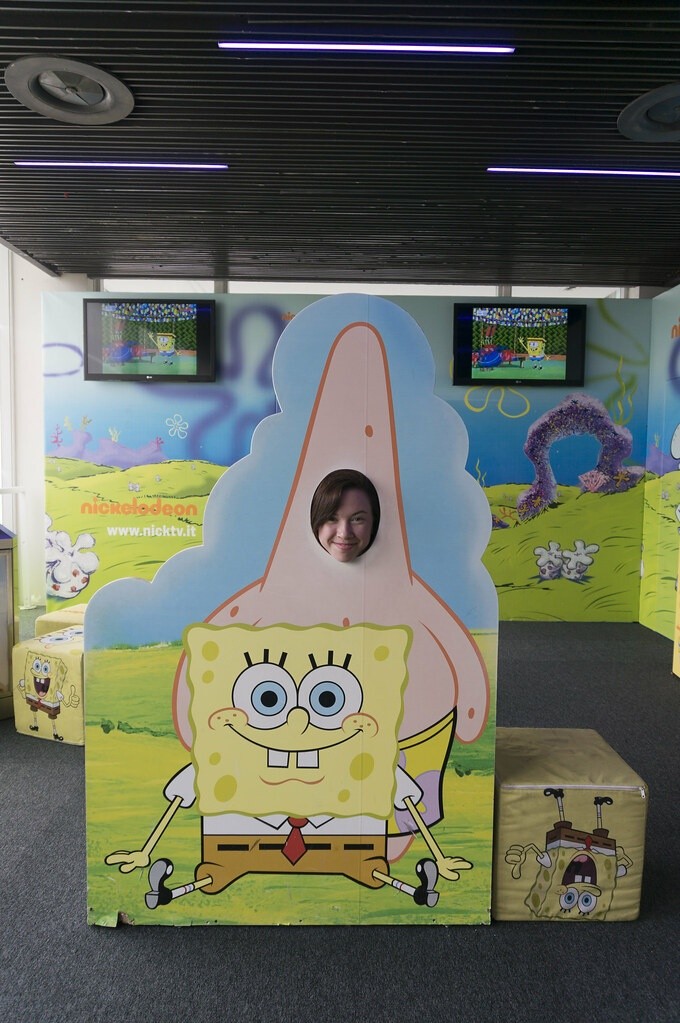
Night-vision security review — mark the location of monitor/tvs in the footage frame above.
[83,298,216,383]
[453,303,587,386]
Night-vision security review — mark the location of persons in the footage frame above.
[311,469,378,562]
